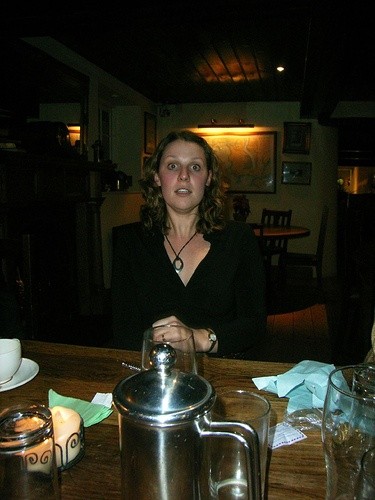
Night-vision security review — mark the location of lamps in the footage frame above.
[198,118,255,129]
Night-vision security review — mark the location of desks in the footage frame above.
[0,338,364,500]
[251,223,312,241]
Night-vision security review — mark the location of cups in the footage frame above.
[141,324,198,376]
[112,344,262,500]
[322,364,374,500]
[48,406,85,468]
[0,405,62,500]
[209,390,270,499]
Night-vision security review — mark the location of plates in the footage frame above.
[0,358,38,392]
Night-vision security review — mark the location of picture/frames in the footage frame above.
[282,121,313,154]
[281,161,313,185]
[99,107,112,158]
[143,111,157,155]
[191,130,277,195]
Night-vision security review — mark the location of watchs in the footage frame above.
[202,328,219,353]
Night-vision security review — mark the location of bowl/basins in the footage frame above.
[0,338,20,385]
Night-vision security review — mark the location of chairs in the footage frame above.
[278,204,330,293]
[260,209,292,259]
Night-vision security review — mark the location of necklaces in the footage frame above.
[164,229,198,273]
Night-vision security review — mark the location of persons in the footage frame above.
[108,132,274,358]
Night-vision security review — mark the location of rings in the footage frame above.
[162,334,165,342]
